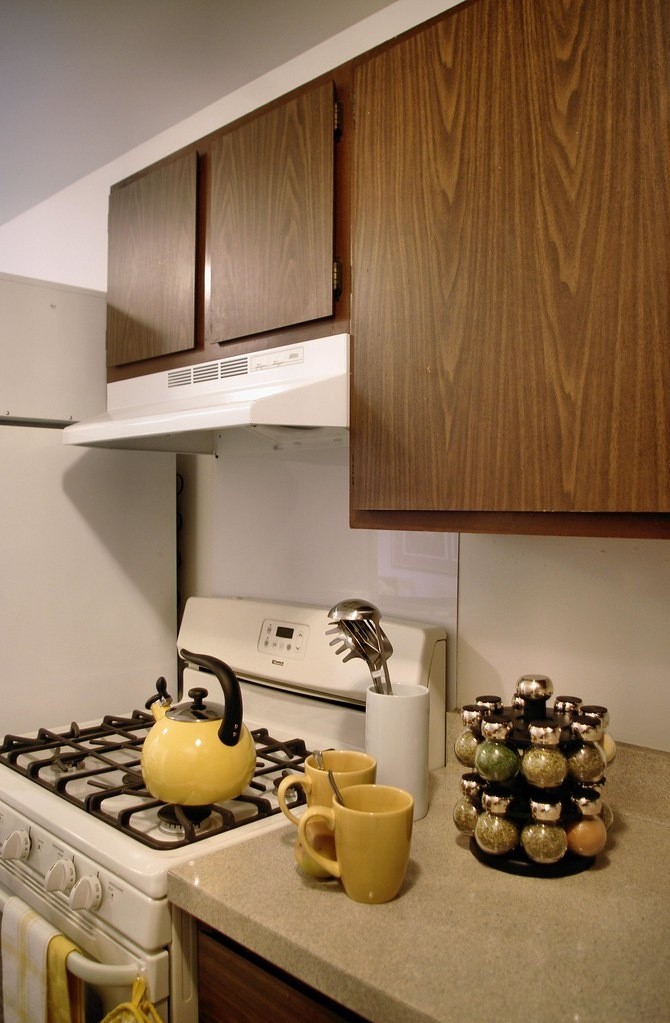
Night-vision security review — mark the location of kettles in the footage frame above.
[143,650,258,807]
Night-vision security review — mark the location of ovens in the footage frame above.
[0,861,172,1023]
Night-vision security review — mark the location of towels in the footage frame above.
[0,895,85,1023]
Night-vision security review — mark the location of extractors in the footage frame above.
[59,333,351,456]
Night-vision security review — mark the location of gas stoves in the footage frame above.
[1,598,452,954]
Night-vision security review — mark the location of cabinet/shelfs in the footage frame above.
[347,0,670,541]
[196,923,369,1023]
[105,66,339,385]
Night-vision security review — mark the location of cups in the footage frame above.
[296,783,414,904]
[360,683,429,822]
[276,750,378,877]
